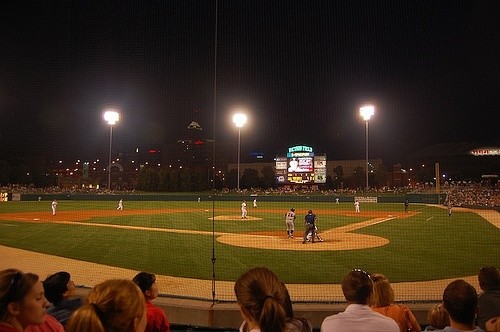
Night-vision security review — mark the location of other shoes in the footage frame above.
[306,239,310,241]
[318,239,323,242]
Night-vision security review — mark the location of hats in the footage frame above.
[291,208,295,211]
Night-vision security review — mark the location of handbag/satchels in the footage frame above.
[398,303,415,332]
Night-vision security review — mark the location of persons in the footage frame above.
[0,182,139,196]
[219,267,313,332]
[214,181,500,207]
[117,198,124,212]
[422,264,500,332]
[52,200,58,217]
[253,198,257,208]
[370,273,421,332]
[303,210,324,242]
[405,198,409,212]
[320,268,399,332]
[354,201,360,214]
[241,200,248,219]
[41,272,81,332]
[0,269,49,332]
[448,202,453,216]
[68,278,146,332]
[284,207,297,239]
[335,197,339,206]
[132,273,171,332]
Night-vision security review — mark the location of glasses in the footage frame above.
[351,269,371,280]
[69,281,74,287]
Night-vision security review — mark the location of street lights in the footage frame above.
[104,110,120,189]
[359,105,375,201]
[232,113,247,192]
